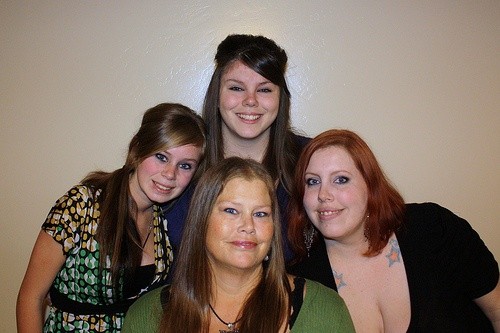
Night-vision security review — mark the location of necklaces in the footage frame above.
[142,223,153,249]
[208,303,241,333]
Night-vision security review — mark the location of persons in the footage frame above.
[161,33,313,261]
[15,103,207,332]
[288,130,499,332]
[121,156,357,333]
